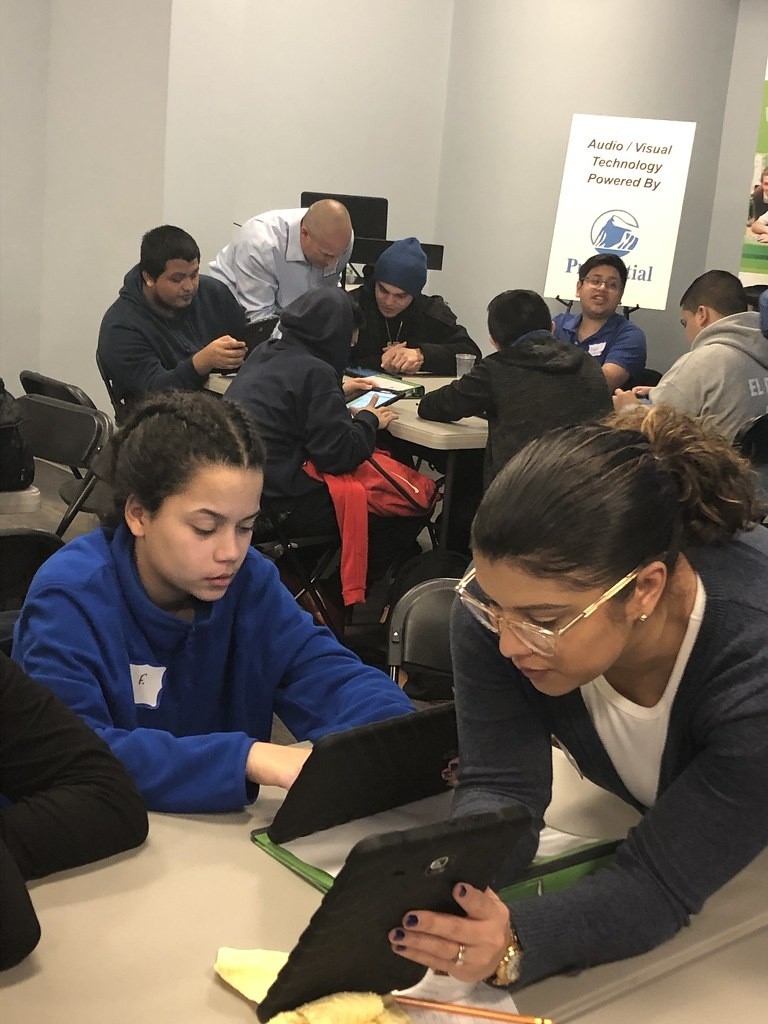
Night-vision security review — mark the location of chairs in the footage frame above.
[1,370,768,703]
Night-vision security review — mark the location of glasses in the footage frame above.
[580,276,624,293]
[453,550,668,658]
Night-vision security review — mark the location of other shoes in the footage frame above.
[292,578,345,641]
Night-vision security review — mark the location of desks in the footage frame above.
[202,366,487,558]
[0,738,768,1024]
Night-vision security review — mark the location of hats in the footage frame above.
[373,237,428,300]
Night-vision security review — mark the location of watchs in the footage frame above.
[490,927,523,986]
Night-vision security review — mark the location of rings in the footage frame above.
[452,943,466,966]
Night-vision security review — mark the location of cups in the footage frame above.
[456,354,477,380]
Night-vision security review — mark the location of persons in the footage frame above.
[221,287,437,645]
[206,199,356,345]
[97,225,258,417]
[0,640,149,970]
[12,391,418,813]
[543,254,646,395]
[387,402,767,987]
[347,236,483,377]
[747,166,768,243]
[610,269,768,463]
[419,288,615,492]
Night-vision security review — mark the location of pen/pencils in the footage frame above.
[394,996,556,1024]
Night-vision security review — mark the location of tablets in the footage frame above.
[256,804,532,1024]
[346,386,405,419]
[221,316,280,376]
[266,702,460,847]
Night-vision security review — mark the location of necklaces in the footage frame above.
[384,316,404,347]
